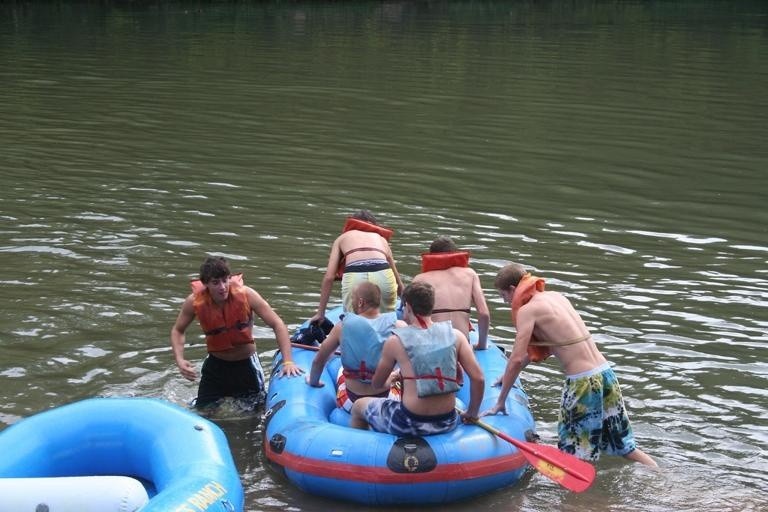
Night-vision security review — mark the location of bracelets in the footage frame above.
[280,360,294,366]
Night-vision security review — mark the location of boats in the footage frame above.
[264,306,538,508]
[0,397,245,512]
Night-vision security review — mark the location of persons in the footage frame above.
[170,255,305,410]
[308,208,405,327]
[480,264,660,469]
[412,236,491,351]
[349,280,485,439]
[306,281,409,415]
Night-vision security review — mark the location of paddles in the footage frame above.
[455,408,596,493]
